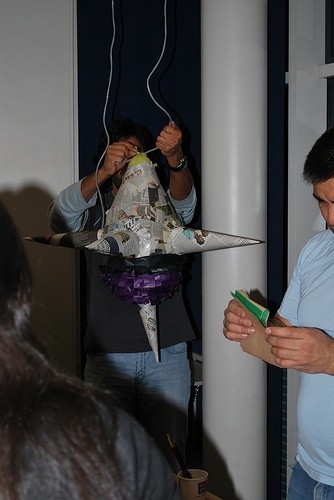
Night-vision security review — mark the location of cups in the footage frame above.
[177,469,208,500]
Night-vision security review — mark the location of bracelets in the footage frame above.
[167,155,187,172]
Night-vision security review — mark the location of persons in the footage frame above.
[0,202,182,500]
[46,121,197,475]
[222,127,334,500]
